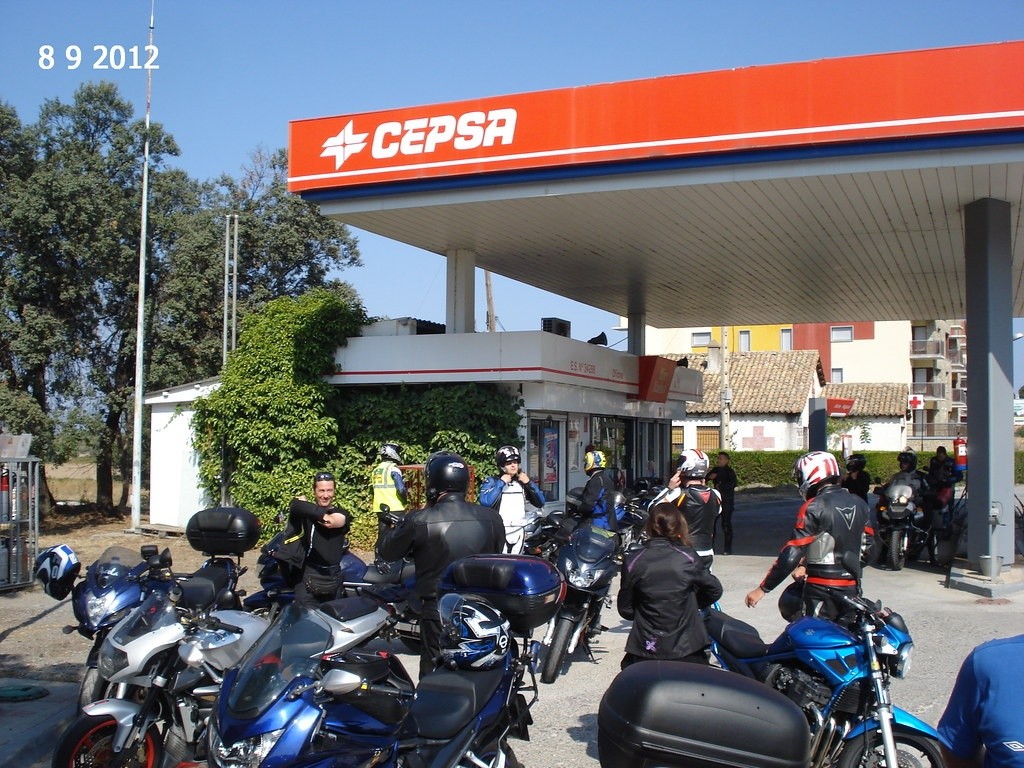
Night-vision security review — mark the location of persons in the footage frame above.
[290,471,350,608]
[905,446,916,456]
[841,454,870,504]
[704,452,738,555]
[480,446,545,555]
[576,450,618,531]
[937,634,1024,768]
[647,450,723,572]
[929,446,964,520]
[370,442,407,537]
[616,502,724,670]
[381,449,506,683]
[882,451,931,492]
[743,451,870,623]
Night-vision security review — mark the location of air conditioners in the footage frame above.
[541,317,571,338]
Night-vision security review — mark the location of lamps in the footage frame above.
[589,332,609,346]
[677,356,689,368]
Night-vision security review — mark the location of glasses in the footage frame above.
[314,474,335,481]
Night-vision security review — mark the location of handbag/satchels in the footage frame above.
[305,564,343,600]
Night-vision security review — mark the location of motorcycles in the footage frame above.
[697,549,954,768]
[206,555,566,768]
[338,536,423,655]
[62,505,265,719]
[874,475,936,572]
[521,476,669,566]
[541,521,648,685]
[51,546,410,768]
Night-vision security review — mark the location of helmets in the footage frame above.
[380,443,404,462]
[425,451,470,494]
[439,600,511,672]
[897,451,917,465]
[33,544,80,600]
[495,446,522,467]
[582,450,606,471]
[846,453,866,472]
[677,449,710,478]
[795,451,839,502]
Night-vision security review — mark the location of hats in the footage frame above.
[937,446,946,453]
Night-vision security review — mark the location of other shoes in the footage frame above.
[930,556,937,565]
[881,559,887,570]
[861,555,867,562]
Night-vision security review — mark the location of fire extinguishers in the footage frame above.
[953,432,968,471]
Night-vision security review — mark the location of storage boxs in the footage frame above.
[185,507,260,554]
[436,550,568,630]
[565,487,583,507]
[597,659,813,768]
